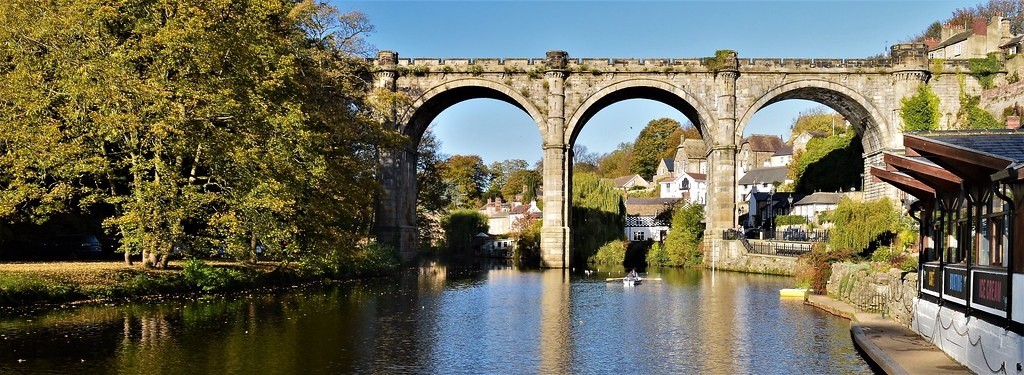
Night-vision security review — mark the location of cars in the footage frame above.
[782,228,806,241]
[37,215,126,260]
[745,228,764,238]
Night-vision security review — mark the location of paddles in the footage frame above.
[638,277,663,281]
[605,275,626,281]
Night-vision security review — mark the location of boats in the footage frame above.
[623,271,643,286]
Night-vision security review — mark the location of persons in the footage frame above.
[731,225,745,235]
[782,226,805,240]
[625,269,638,281]
